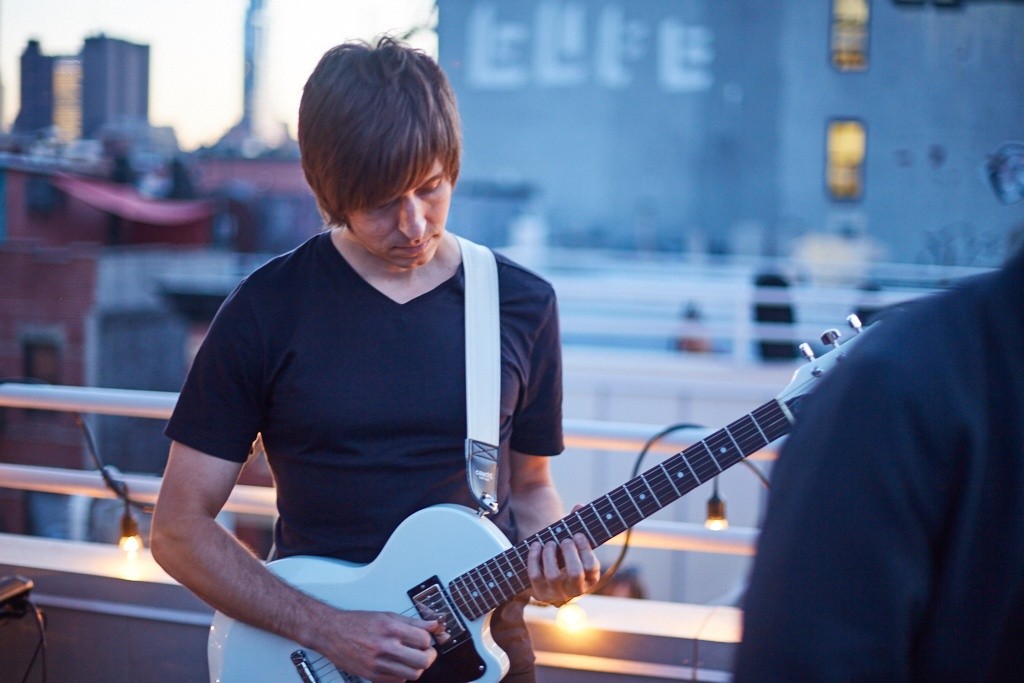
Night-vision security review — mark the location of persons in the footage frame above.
[150,37,600,683]
[737,137,1024,683]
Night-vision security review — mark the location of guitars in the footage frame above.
[207,315,884,683]
